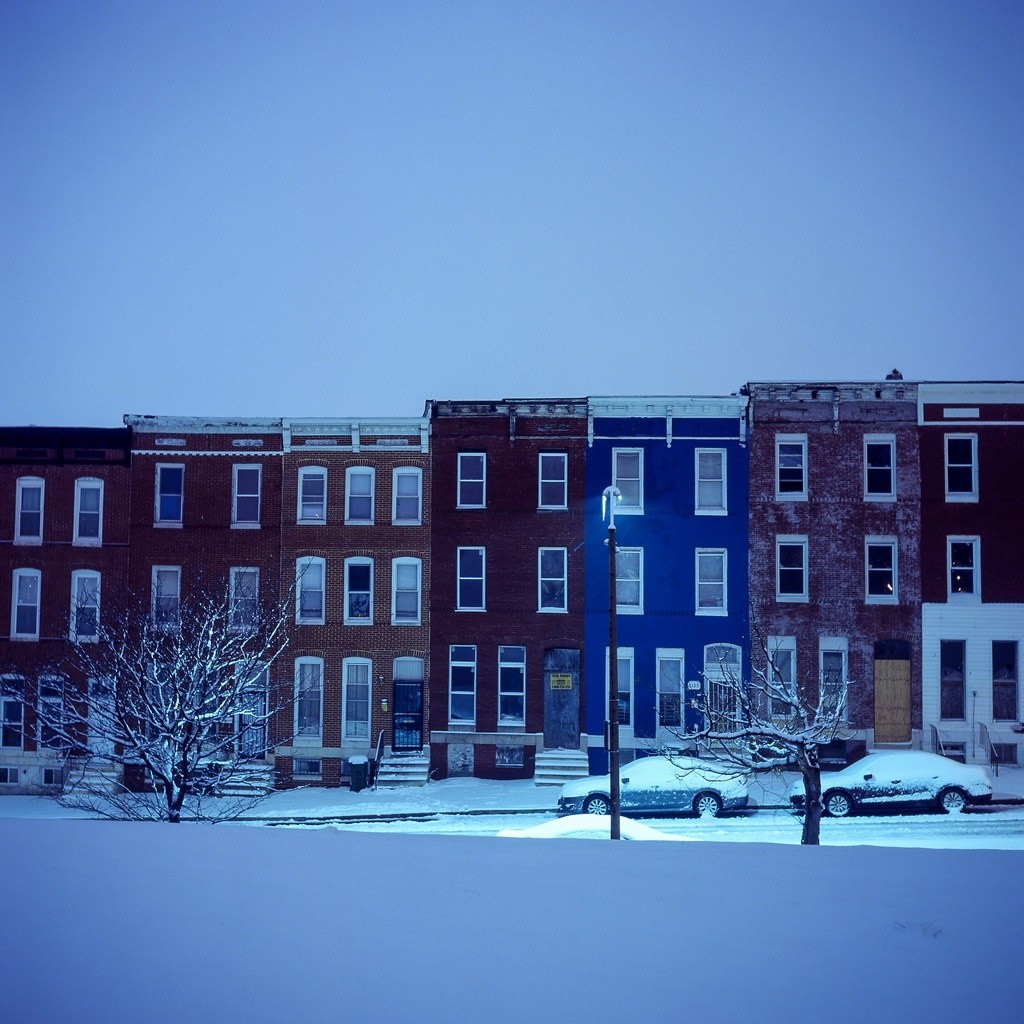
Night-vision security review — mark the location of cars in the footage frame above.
[789,751,993,818]
[557,756,748,819]
[498,815,687,840]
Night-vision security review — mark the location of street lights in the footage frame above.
[602,485,622,839]
